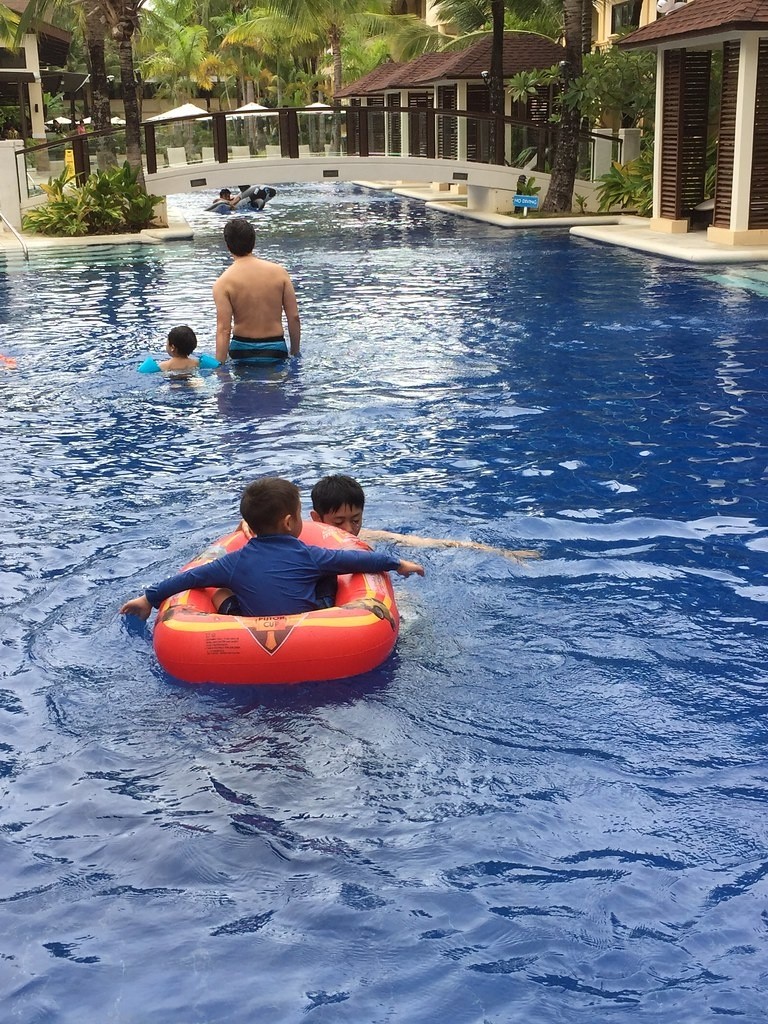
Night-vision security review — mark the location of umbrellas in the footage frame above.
[225,101,279,156]
[145,102,211,145]
[76,116,92,125]
[111,116,126,124]
[295,102,346,148]
[44,115,71,125]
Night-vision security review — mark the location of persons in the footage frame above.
[118,476,424,617]
[309,474,365,537]
[76,119,85,134]
[0,116,32,140]
[43,119,62,133]
[158,325,199,371]
[212,188,242,210]
[211,218,301,365]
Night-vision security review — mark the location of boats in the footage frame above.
[152,519,399,685]
